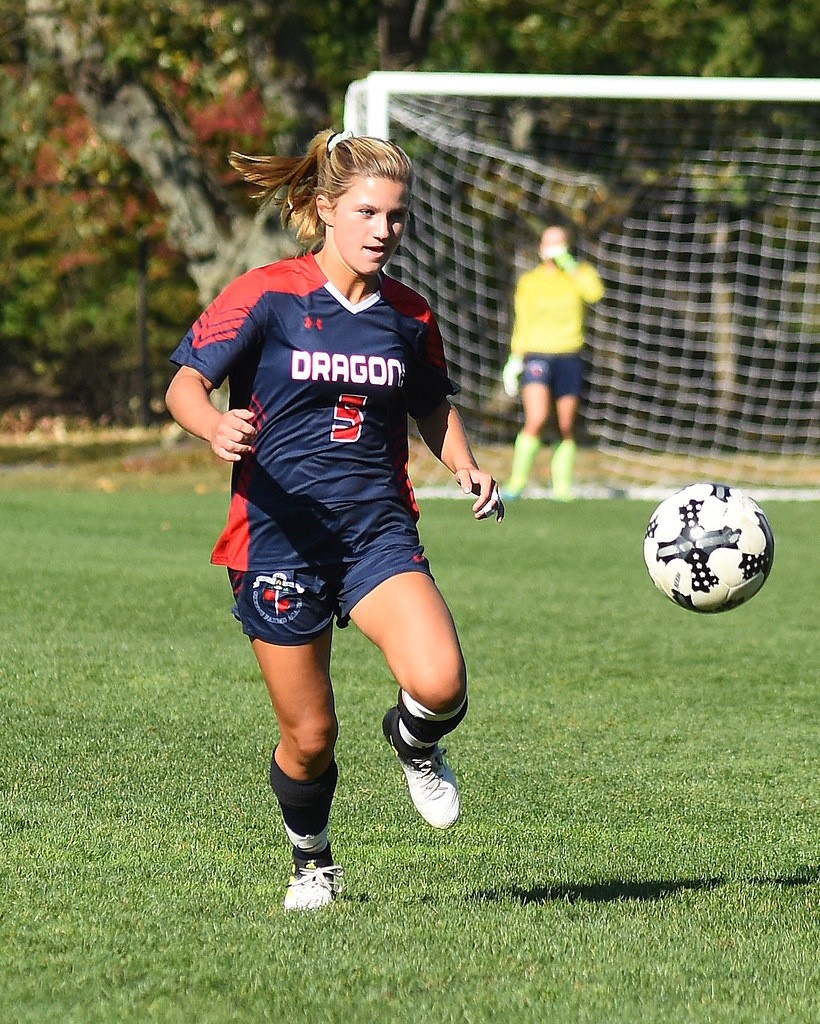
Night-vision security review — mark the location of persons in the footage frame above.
[502,226,606,505]
[162,128,513,909]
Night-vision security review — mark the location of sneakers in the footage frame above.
[284,854,342,911]
[382,707,459,829]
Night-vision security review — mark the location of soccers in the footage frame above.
[644,483,775,615]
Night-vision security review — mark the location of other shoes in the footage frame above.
[499,481,524,501]
